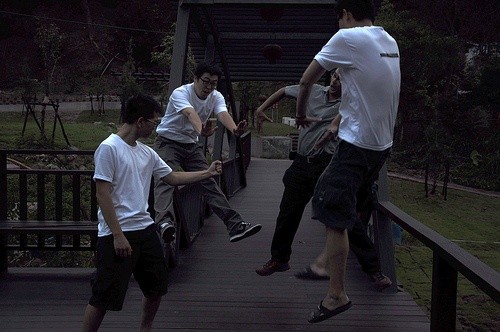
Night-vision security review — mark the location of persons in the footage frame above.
[154,61,262,243]
[84,93,222,332]
[256,0,401,325]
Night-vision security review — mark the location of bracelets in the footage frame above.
[295,114,306,119]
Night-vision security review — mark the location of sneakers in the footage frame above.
[230,221,262,243]
[158,222,176,244]
[369,272,392,290]
[256,258,290,276]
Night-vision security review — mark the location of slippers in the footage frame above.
[307,299,352,323]
[295,265,330,280]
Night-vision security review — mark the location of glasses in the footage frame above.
[330,71,340,81]
[199,77,218,89]
[144,118,161,127]
